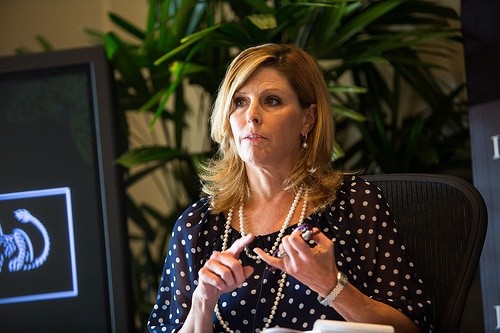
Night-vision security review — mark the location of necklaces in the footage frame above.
[214,183,308,333]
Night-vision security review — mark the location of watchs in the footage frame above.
[319,271,348,306]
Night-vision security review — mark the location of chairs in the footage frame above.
[356,173,489,333]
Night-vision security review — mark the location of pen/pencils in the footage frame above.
[272,223,312,257]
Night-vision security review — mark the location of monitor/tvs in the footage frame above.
[0,46,137,333]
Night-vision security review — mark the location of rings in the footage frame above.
[280,251,287,258]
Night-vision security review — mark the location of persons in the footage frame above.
[146,43,434,333]
